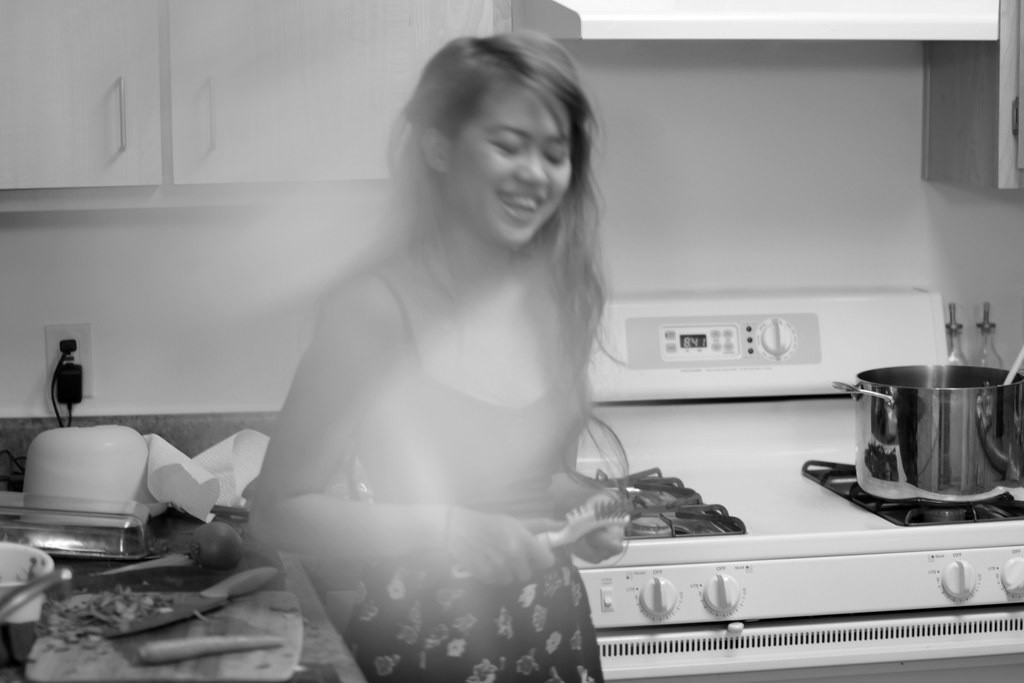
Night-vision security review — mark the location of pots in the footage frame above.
[831,364,1024,507]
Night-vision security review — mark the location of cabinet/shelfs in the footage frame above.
[921,0,1024,194]
[0,0,513,225]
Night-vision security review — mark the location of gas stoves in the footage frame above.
[565,289,1024,630]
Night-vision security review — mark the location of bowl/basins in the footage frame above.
[0,540,55,606]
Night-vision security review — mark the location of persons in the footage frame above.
[244,30,631,683]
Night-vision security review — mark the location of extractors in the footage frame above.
[514,0,1001,44]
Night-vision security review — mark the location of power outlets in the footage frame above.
[43,322,94,400]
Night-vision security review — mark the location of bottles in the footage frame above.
[973,302,1005,369]
[945,302,968,367]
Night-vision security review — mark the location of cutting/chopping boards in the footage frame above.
[24,556,304,683]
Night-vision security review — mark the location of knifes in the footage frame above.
[102,567,278,639]
